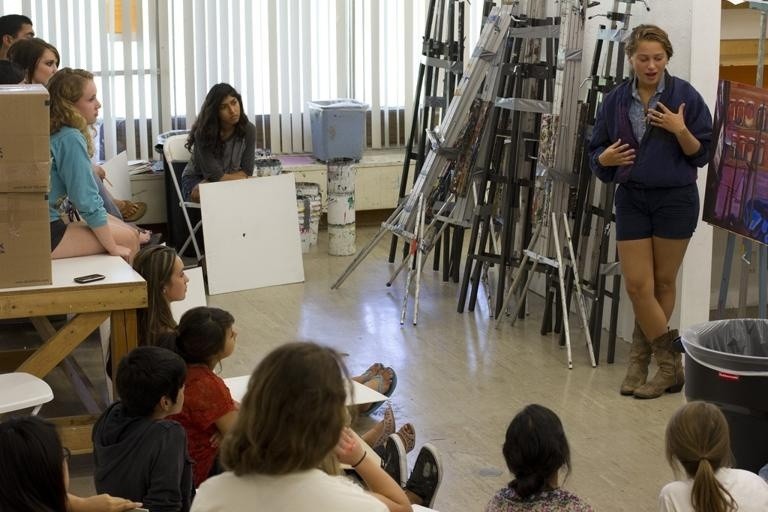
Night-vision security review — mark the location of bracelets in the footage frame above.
[246,175,249,179]
[351,451,367,468]
[676,128,688,141]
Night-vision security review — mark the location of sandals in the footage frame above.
[120,200,147,222]
[396,423,415,454]
[138,228,162,249]
[372,406,395,449]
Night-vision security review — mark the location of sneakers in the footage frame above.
[381,433,408,488]
[401,443,444,509]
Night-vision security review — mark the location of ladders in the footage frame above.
[331,0,635,370]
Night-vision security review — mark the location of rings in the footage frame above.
[659,113,663,118]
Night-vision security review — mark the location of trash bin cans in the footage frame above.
[681,316,768,475]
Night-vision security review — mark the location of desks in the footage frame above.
[0,254,150,459]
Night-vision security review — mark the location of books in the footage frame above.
[129,160,151,176]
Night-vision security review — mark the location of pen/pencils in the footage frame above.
[104,177,112,187]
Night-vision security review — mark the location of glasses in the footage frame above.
[64,447,72,463]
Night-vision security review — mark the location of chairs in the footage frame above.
[163,134,204,267]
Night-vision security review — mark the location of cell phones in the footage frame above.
[74,272,107,284]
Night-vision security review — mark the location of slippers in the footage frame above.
[359,367,397,417]
[362,363,383,382]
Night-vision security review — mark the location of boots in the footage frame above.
[633,329,684,398]
[621,318,651,395]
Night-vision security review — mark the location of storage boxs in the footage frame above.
[1,80,51,196]
[1,194,53,286]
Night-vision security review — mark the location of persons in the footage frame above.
[7,39,162,249]
[0,415,144,512]
[47,67,140,265]
[659,400,768,512]
[482,405,594,512]
[190,342,413,510]
[172,308,414,487]
[93,346,442,512]
[180,84,256,277]
[108,246,396,417]
[0,15,147,222]
[588,24,712,399]
